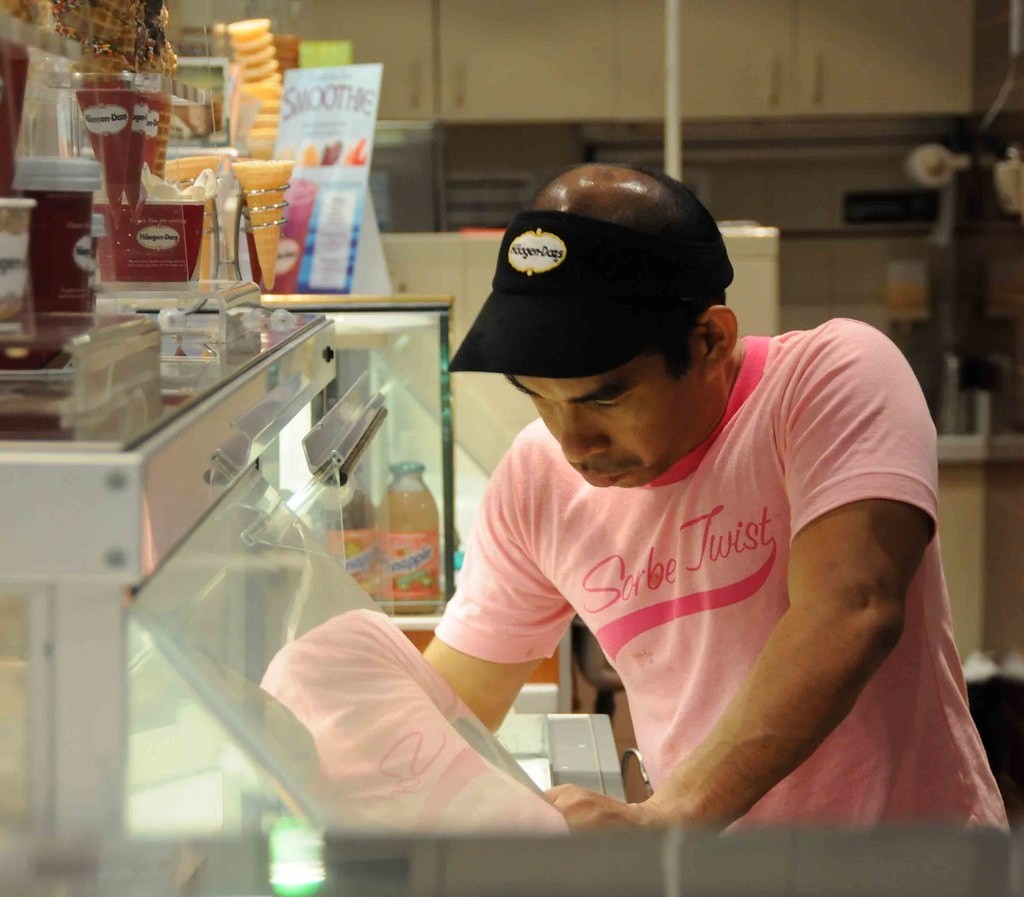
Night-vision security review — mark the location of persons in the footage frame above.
[421,163,1010,837]
[258,605,573,836]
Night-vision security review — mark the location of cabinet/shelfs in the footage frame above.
[167,0,977,141]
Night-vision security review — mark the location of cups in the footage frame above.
[258,180,319,294]
[0,198,37,321]
[12,157,101,329]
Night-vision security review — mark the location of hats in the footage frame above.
[447,168,733,378]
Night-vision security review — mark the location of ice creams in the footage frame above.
[46,0,178,181]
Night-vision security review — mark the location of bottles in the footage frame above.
[323,481,379,607]
[374,461,441,614]
